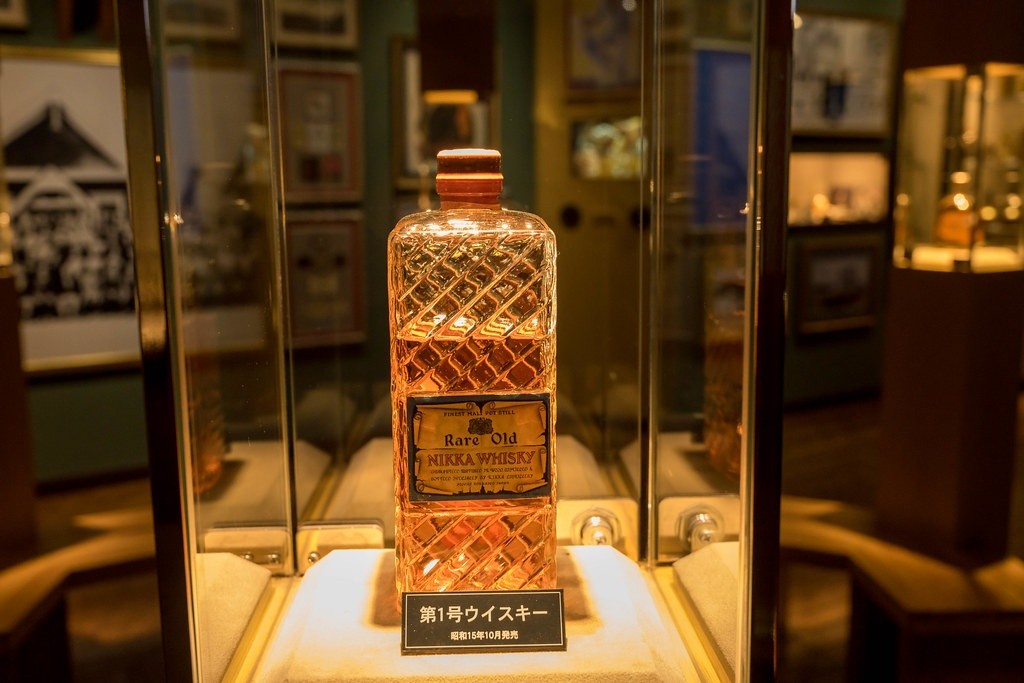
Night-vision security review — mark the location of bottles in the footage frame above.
[388,148,557,611]
[936,171,982,246]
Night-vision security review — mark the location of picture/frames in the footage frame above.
[1,46,261,374]
[272,1,356,49]
[561,2,653,105]
[791,232,890,337]
[282,210,367,347]
[160,0,241,40]
[391,36,500,190]
[787,11,901,133]
[270,55,368,204]
